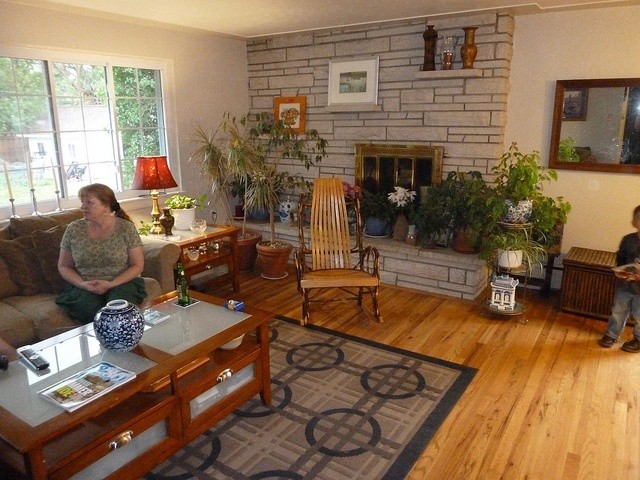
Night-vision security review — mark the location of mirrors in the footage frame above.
[548,77,640,175]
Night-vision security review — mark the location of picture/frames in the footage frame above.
[328,55,379,106]
[273,96,306,134]
[562,88,587,122]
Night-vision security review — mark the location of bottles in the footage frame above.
[188,250,199,261]
[176,263,190,304]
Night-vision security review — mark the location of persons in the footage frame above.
[600,206,639,352]
[57,184,147,325]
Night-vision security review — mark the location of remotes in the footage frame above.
[16,344,50,371]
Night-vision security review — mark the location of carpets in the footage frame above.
[142,315,480,480]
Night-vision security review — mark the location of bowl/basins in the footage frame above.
[190,219,207,233]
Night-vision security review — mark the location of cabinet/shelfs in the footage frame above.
[494,218,564,296]
[559,247,640,326]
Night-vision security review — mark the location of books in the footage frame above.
[36,360,136,414]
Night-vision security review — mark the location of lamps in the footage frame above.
[129,156,179,233]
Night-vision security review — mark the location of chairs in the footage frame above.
[293,177,384,326]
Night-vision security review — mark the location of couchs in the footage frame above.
[0,208,182,362]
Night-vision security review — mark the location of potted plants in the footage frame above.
[227,173,252,221]
[183,110,262,270]
[164,192,210,230]
[425,170,497,253]
[485,141,568,221]
[276,176,301,224]
[242,165,293,279]
[481,231,549,280]
[362,198,394,240]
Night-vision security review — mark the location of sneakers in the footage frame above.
[622,337,640,353]
[599,335,617,346]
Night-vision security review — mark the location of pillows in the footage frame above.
[10,214,67,237]
[0,235,41,296]
[33,224,68,294]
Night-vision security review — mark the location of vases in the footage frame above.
[406,225,418,244]
[393,216,408,241]
[95,299,144,353]
[489,274,519,314]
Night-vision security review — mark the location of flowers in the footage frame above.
[387,186,417,207]
